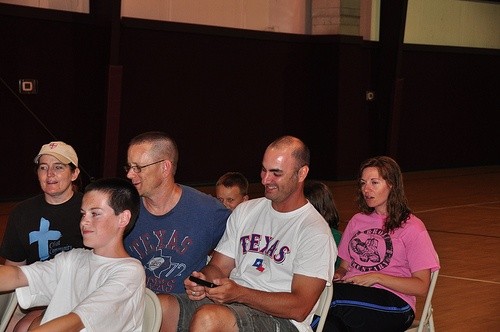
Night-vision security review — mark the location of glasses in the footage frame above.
[124,159,172,173]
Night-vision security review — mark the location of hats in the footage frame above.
[35,142,78,166]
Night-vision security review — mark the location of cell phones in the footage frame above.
[188,276,215,288]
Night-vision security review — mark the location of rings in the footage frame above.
[192,292,196,296]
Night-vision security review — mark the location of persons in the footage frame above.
[303,181,343,271]
[122,130,233,294]
[156,134,338,332]
[0,177,147,332]
[215,172,249,212]
[0,141,92,332]
[310,156,440,332]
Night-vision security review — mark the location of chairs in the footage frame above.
[404,269,439,332]
